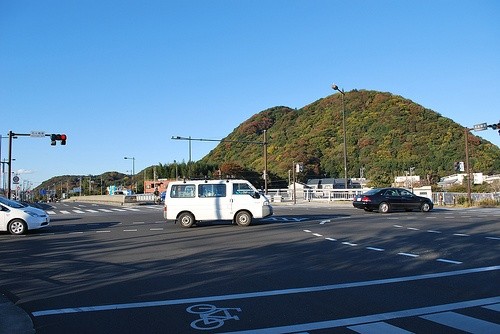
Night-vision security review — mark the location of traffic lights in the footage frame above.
[492,123,500,130]
[51,134,67,141]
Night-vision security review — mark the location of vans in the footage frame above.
[163,174,274,228]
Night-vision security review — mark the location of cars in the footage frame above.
[0,196,51,235]
[352,187,434,213]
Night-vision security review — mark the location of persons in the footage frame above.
[234,186,241,194]
[154,188,160,204]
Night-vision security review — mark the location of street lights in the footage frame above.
[123,156,136,191]
[2,158,16,194]
[331,84,348,190]
